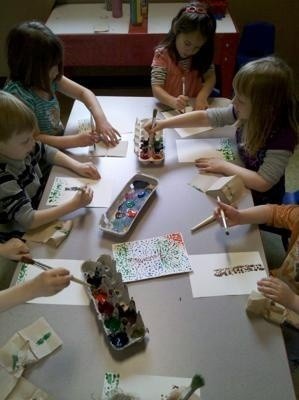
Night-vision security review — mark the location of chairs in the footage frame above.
[256,183,299,256]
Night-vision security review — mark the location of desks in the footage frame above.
[2,94,296,400]
[44,0,240,102]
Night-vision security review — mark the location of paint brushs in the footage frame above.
[90,113,97,154]
[20,256,98,290]
[179,76,186,116]
[169,374,206,399]
[145,107,158,161]
[76,187,88,194]
[190,194,237,236]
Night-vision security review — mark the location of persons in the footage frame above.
[1,20,122,147]
[1,235,73,313]
[140,55,299,207]
[149,0,217,112]
[213,200,299,373]
[0,91,101,229]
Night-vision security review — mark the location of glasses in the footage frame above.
[185,6,207,14]
